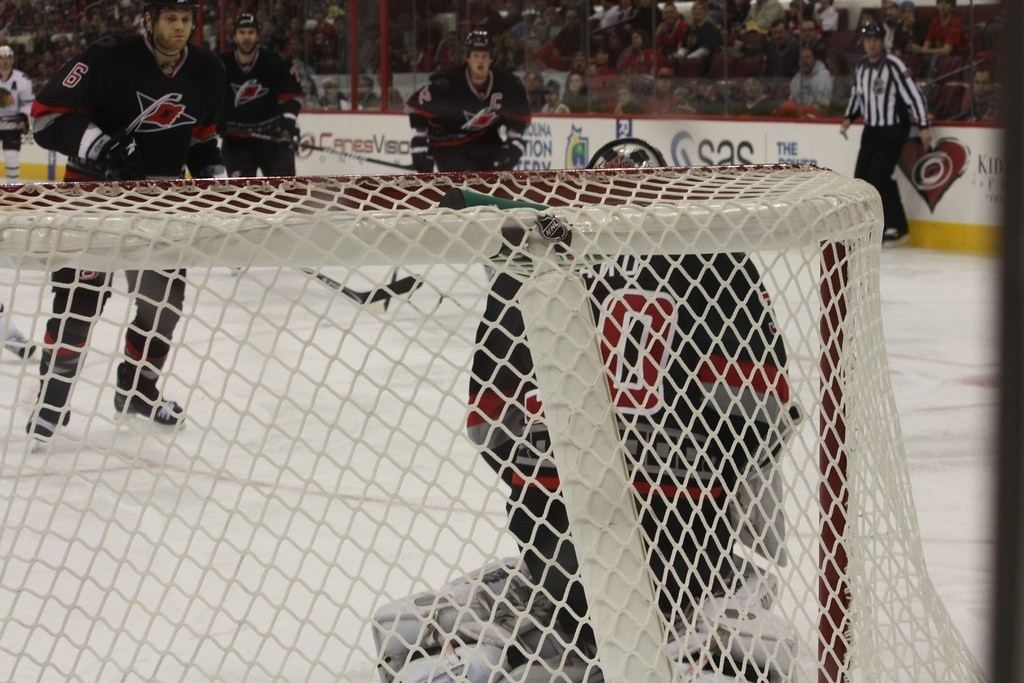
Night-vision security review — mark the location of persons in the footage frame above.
[25,0,236,443]
[0,0,1003,125]
[0,302,36,360]
[406,28,532,174]
[0,44,36,185]
[373,138,798,683]
[839,20,932,249]
[216,13,306,178]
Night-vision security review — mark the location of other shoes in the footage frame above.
[884,227,909,246]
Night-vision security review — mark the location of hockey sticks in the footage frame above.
[228,127,418,171]
[296,264,425,305]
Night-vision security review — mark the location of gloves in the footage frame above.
[87,132,142,177]
[411,135,435,173]
[487,137,528,171]
[282,113,300,152]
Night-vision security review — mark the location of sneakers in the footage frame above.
[115,362,187,433]
[25,362,71,453]
[0,303,35,360]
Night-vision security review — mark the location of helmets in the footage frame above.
[467,30,495,52]
[0,46,14,57]
[588,139,667,169]
[860,20,886,37]
[143,0,199,14]
[231,13,262,38]
